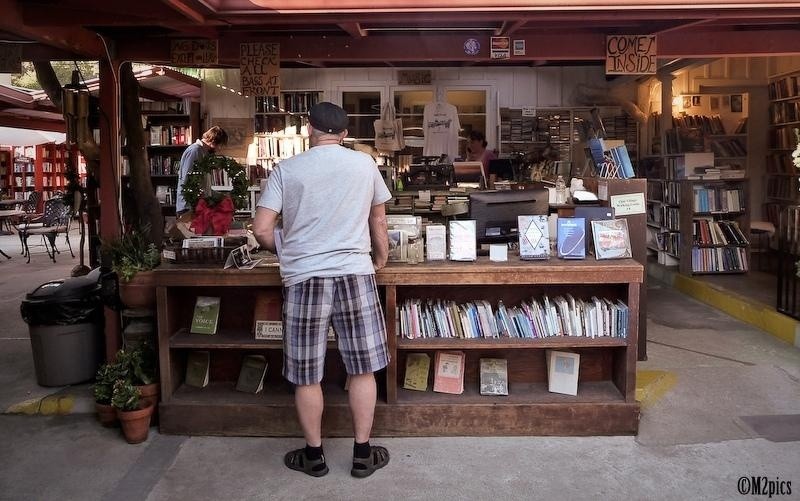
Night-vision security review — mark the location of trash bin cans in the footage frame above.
[20,266,103,388]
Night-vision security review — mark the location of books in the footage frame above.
[0,75,799,395]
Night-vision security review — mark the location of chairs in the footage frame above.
[24,204,74,263]
[18,198,60,257]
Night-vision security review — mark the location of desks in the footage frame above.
[0,210,26,259]
[0,199,27,234]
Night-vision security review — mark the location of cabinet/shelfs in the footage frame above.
[643,67,800,277]
[211,184,260,218]
[253,89,324,187]
[0,142,87,220]
[155,246,643,436]
[498,106,641,178]
[511,180,648,362]
[121,101,200,216]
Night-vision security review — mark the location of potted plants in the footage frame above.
[110,377,154,444]
[117,332,161,408]
[91,363,132,429]
[98,217,161,311]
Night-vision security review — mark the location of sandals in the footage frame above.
[349,445,391,478]
[283,448,330,477]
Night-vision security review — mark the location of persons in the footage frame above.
[252,102,393,478]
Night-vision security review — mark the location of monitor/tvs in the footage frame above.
[489,159,516,180]
[469,188,549,256]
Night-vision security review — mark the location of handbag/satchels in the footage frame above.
[373,101,406,152]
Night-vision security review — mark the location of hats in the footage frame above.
[308,101,350,134]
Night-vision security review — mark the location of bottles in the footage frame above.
[555,175,566,204]
